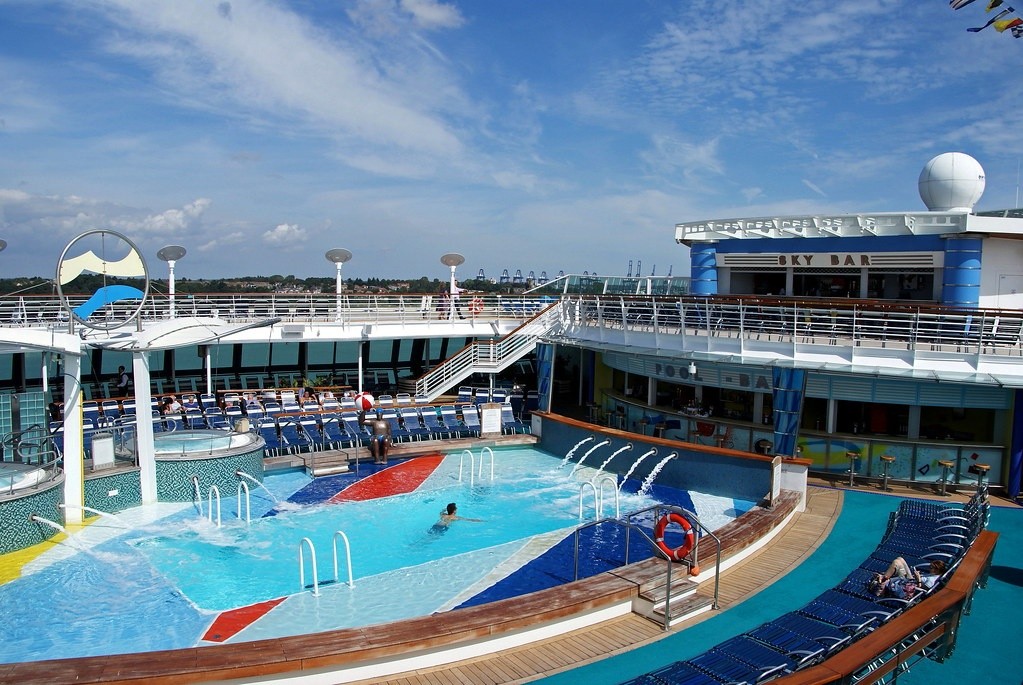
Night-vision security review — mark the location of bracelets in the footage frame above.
[918,581,922,583]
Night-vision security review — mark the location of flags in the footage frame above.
[950,0,1023,39]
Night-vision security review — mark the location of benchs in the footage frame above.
[587,302,1022,356]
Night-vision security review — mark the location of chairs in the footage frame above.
[502,298,556,314]
[0,299,332,327]
[619,486,992,685]
[47,371,532,459]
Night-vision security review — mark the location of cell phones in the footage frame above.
[914,566,917,572]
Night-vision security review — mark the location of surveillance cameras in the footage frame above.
[687,362,696,374]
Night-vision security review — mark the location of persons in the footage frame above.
[116,365,129,412]
[347,391,357,397]
[436,284,450,319]
[880,557,942,590]
[246,393,258,405]
[321,392,336,404]
[447,503,458,518]
[447,280,466,319]
[159,396,182,419]
[182,396,200,415]
[359,407,391,465]
[300,391,315,405]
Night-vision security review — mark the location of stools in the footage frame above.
[638,419,650,435]
[713,434,726,448]
[842,452,861,487]
[761,440,773,454]
[691,430,703,444]
[603,410,615,429]
[933,460,954,497]
[655,424,668,437]
[586,400,602,424]
[971,464,991,499]
[797,446,804,454]
[615,413,626,430]
[875,456,896,491]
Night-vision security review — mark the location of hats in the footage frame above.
[376,408,383,413]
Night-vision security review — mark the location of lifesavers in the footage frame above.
[653,513,695,561]
[469,298,484,314]
